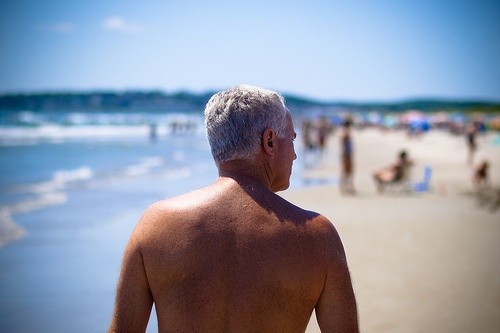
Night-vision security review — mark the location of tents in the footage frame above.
[324,105,500,132]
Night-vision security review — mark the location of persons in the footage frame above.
[106,84,361,333]
[302,110,489,194]
